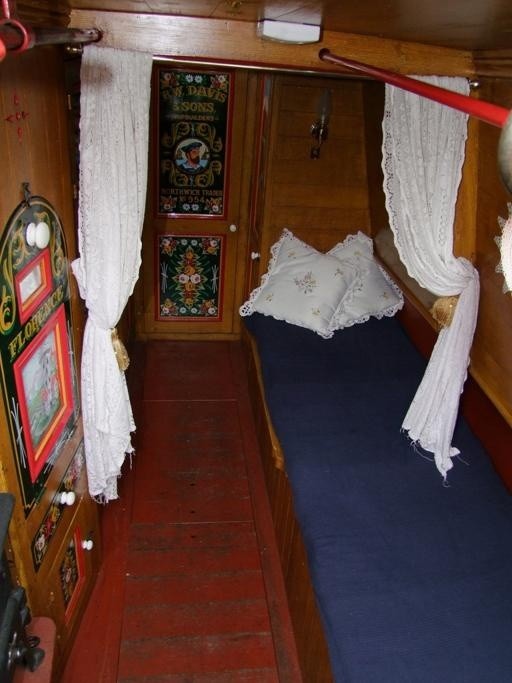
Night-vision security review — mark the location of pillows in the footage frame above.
[322,230,405,331]
[239,228,370,336]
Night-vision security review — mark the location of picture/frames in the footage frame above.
[13,303,76,485]
[14,247,55,326]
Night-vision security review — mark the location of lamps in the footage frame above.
[307,100,331,158]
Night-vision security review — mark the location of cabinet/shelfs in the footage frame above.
[0,183,98,636]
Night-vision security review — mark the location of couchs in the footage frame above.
[240,311,512,682]
